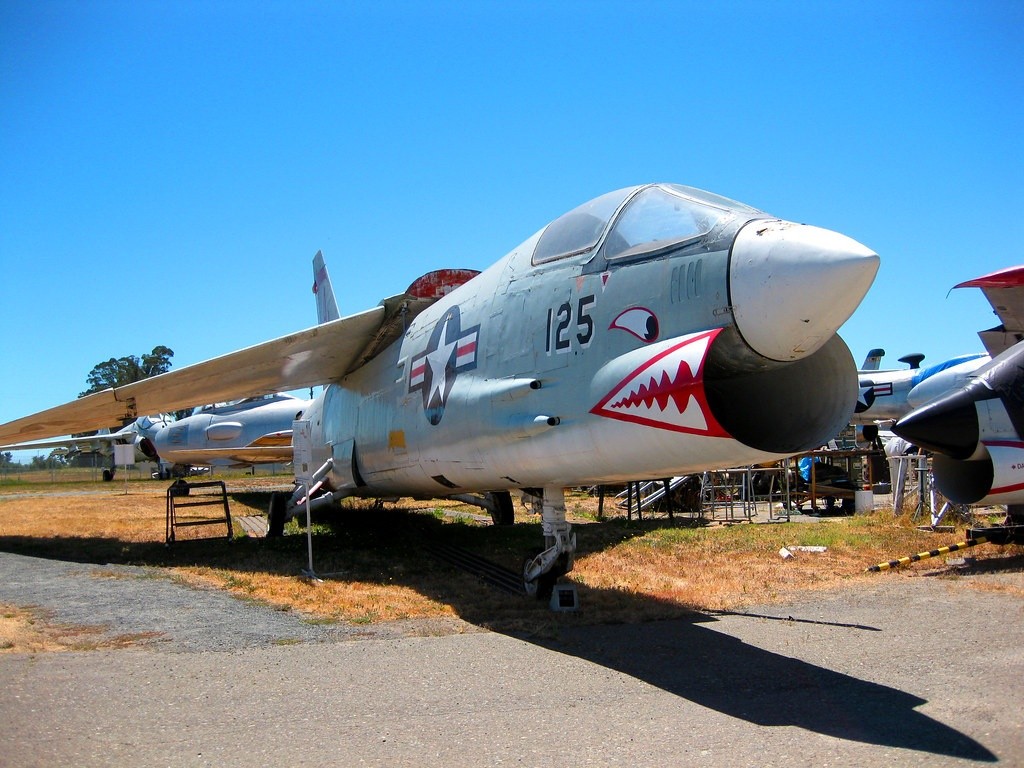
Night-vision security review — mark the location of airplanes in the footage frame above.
[0,389,315,497]
[836,265,1024,545]
[0,182,881,607]
